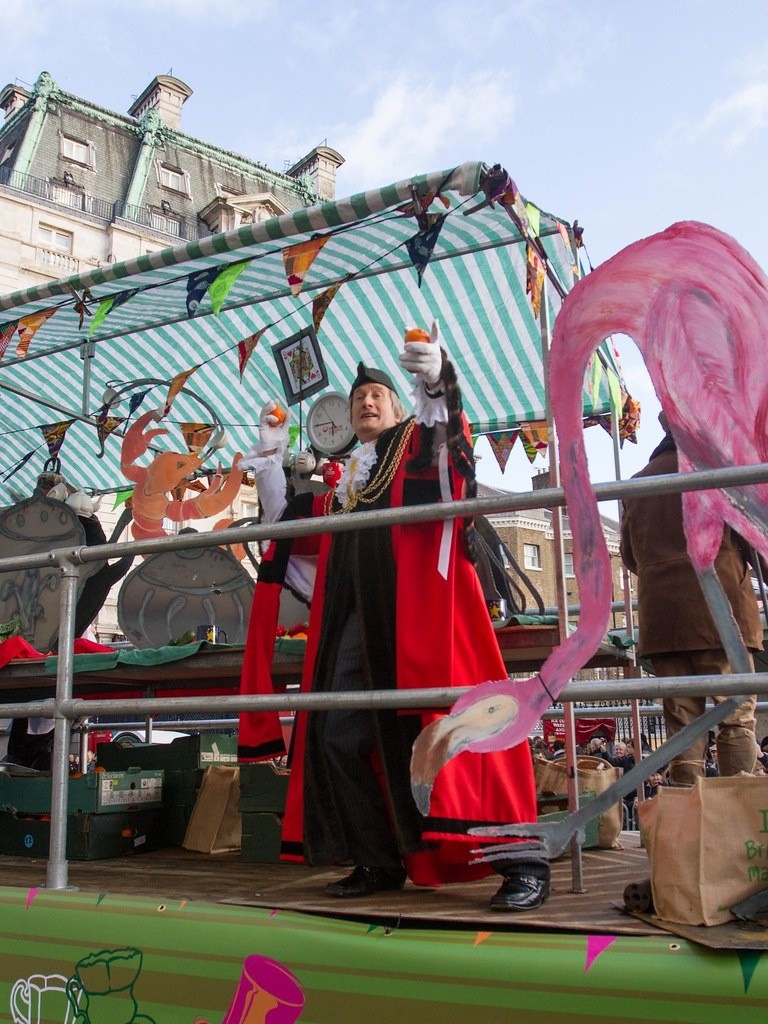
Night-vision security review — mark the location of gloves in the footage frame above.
[399,317,442,383]
[259,397,291,452]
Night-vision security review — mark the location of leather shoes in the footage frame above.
[327,865,404,897]
[489,873,550,911]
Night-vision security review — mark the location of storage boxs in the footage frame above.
[0,733,290,863]
[537,790,601,855]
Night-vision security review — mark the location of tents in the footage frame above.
[0,163,652,895]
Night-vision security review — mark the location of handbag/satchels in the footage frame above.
[635,767,767,926]
[182,764,243,854]
[531,757,626,850]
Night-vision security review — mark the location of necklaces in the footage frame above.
[323,419,414,516]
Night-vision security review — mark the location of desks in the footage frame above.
[0,628,645,892]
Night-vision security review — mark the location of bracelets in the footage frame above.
[423,385,445,399]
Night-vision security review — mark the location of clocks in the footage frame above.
[306,391,358,454]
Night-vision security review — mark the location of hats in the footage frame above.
[349,359,400,396]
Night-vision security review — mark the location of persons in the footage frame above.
[526,729,768,831]
[238,318,552,910]
[619,409,768,787]
[68,751,98,772]
